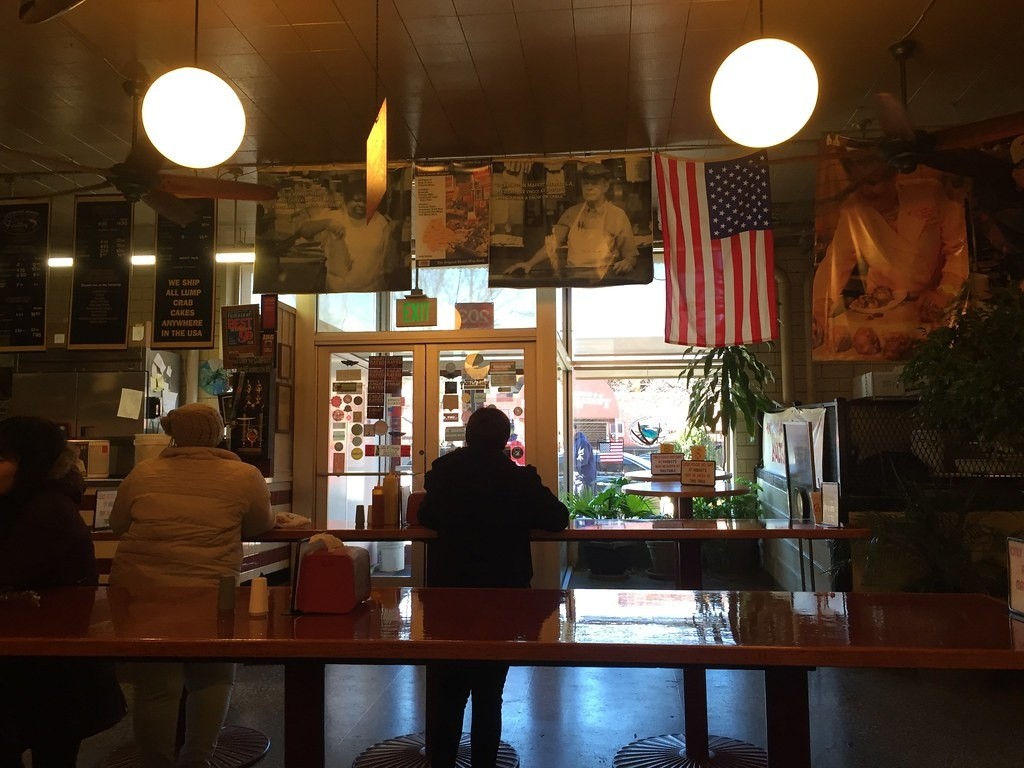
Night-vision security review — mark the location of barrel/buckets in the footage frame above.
[376,541,412,572]
[133,434,175,467]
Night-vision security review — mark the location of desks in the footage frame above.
[0,585,1024,768]
[623,468,733,589]
[89,516,874,768]
[620,481,751,590]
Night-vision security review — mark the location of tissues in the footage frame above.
[297,533,372,615]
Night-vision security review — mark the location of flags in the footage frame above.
[651,151,778,347]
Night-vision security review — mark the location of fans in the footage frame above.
[748,39,1024,214]
[1,79,279,228]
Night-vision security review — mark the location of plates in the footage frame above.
[849,289,907,313]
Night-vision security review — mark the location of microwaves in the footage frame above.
[67,440,109,478]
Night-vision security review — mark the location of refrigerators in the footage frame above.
[11,350,186,437]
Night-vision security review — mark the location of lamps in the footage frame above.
[47,257,74,268]
[132,255,158,265]
[141,1,246,170]
[214,167,255,264]
[710,0,820,150]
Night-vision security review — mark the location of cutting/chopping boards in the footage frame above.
[812,300,957,361]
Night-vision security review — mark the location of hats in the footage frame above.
[160,403,224,447]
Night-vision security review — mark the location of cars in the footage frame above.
[572,451,651,498]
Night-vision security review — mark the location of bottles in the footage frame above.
[383,475,398,524]
[372,486,384,529]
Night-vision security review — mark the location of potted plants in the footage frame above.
[632,509,677,582]
[560,476,659,582]
[694,475,764,582]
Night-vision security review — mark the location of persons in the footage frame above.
[109,402,275,768]
[505,165,640,277]
[812,147,970,347]
[418,405,569,768]
[299,176,393,293]
[0,415,130,768]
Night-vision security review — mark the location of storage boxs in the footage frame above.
[852,371,906,397]
[1006,529,1024,617]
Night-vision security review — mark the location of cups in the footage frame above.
[218,576,234,609]
[355,505,365,529]
[971,274,989,295]
[367,506,373,527]
[249,577,268,612]
[809,492,822,523]
